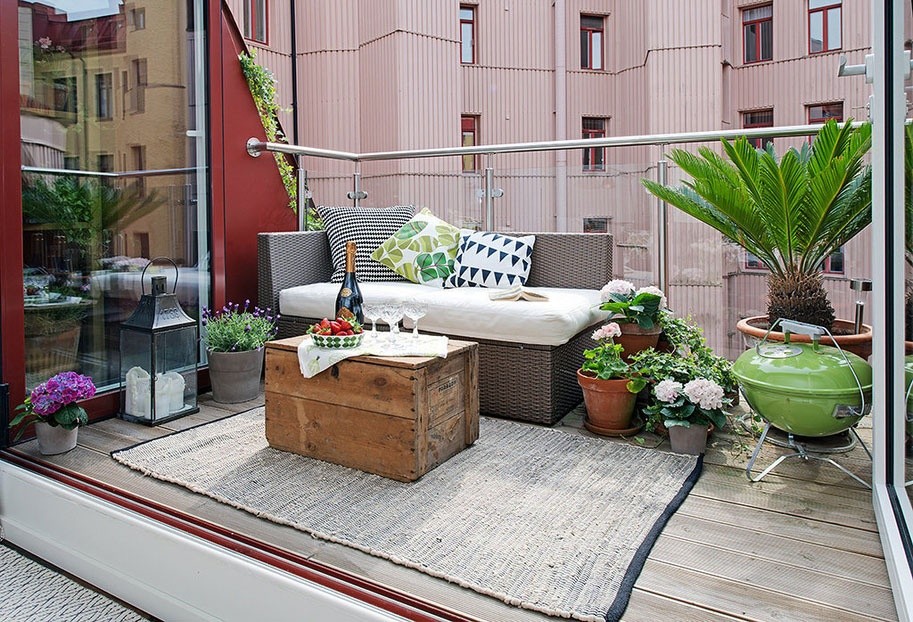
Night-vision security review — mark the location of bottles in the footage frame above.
[334,241,362,327]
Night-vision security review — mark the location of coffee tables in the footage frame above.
[265,334,479,484]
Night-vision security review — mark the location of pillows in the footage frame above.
[444,228,536,287]
[369,208,475,286]
[316,203,419,282]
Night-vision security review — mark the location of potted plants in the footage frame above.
[640,117,876,446]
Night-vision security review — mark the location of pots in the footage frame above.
[730,319,872,438]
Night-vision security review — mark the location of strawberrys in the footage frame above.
[306,315,364,336]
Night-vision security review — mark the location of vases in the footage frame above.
[35,412,79,456]
[617,325,662,355]
[200,345,264,403]
[662,421,708,456]
[577,367,644,435]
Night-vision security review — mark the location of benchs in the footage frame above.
[256,232,615,427]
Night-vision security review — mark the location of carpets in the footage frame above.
[110,405,703,622]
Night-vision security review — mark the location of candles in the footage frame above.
[124,367,184,420]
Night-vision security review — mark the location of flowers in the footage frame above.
[597,280,667,332]
[8,372,94,443]
[582,325,647,395]
[653,379,730,425]
[199,301,280,354]
